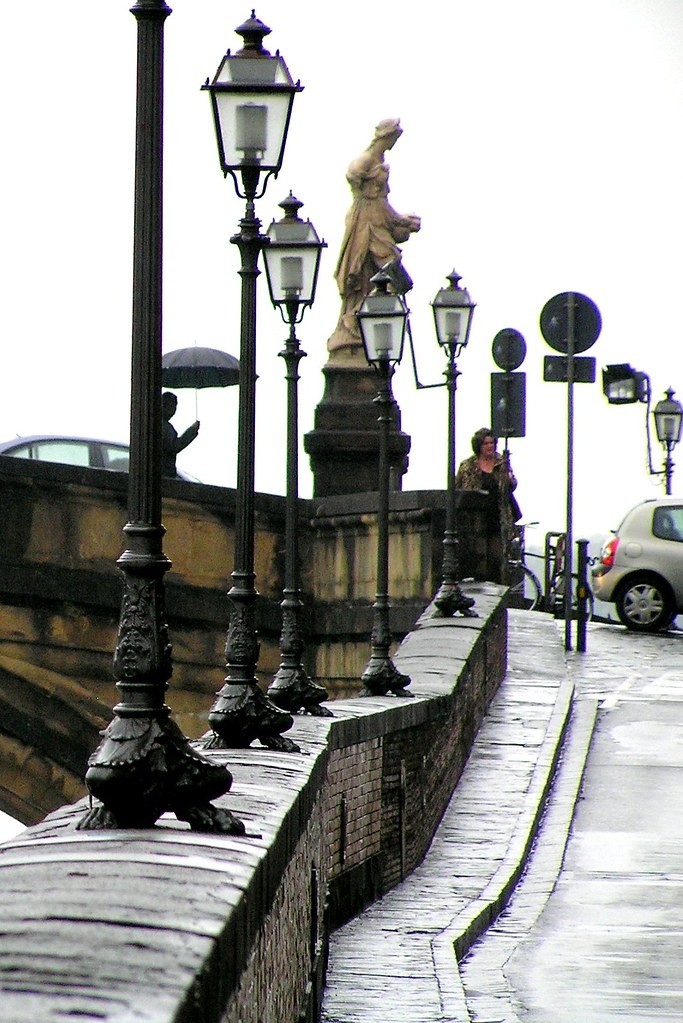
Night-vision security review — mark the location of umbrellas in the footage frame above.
[161,347,260,420]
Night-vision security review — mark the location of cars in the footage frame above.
[0,435,203,484]
[591,498,683,633]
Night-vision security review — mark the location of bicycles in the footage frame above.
[502,522,595,622]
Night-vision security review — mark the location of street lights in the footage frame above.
[351,264,412,700]
[260,188,336,721]
[597,360,683,494]
[427,267,480,620]
[199,8,303,756]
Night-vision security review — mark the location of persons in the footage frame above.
[456,427,518,495]
[162,392,200,479]
[326,117,421,351]
